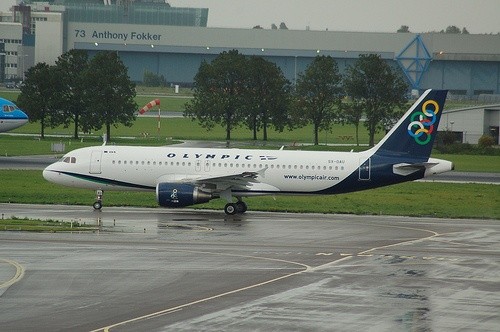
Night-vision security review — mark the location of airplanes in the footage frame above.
[44,89,455,218]
[0,97,28,136]
